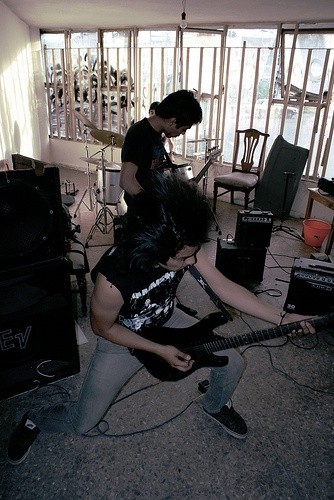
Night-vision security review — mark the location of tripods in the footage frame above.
[73,128,117,249]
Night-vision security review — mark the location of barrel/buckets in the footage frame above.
[303,218,331,246]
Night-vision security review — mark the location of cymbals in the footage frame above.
[70,108,98,130]
[80,157,112,167]
[90,130,126,148]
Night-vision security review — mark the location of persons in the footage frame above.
[119,90,202,207]
[4,176,321,464]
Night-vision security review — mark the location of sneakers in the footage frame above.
[35,360,60,378]
[6,409,42,466]
[203,398,249,439]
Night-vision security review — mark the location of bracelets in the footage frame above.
[279,312,287,325]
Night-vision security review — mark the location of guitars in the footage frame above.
[130,312,334,382]
[195,145,222,183]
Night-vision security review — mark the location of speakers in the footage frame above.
[0,166,67,273]
[234,210,273,247]
[0,257,81,402]
[283,255,334,317]
[214,236,267,283]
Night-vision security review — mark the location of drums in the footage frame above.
[116,190,129,217]
[177,162,194,180]
[97,163,124,206]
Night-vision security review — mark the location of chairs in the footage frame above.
[213,129,270,214]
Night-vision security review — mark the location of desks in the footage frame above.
[302,187,334,255]
